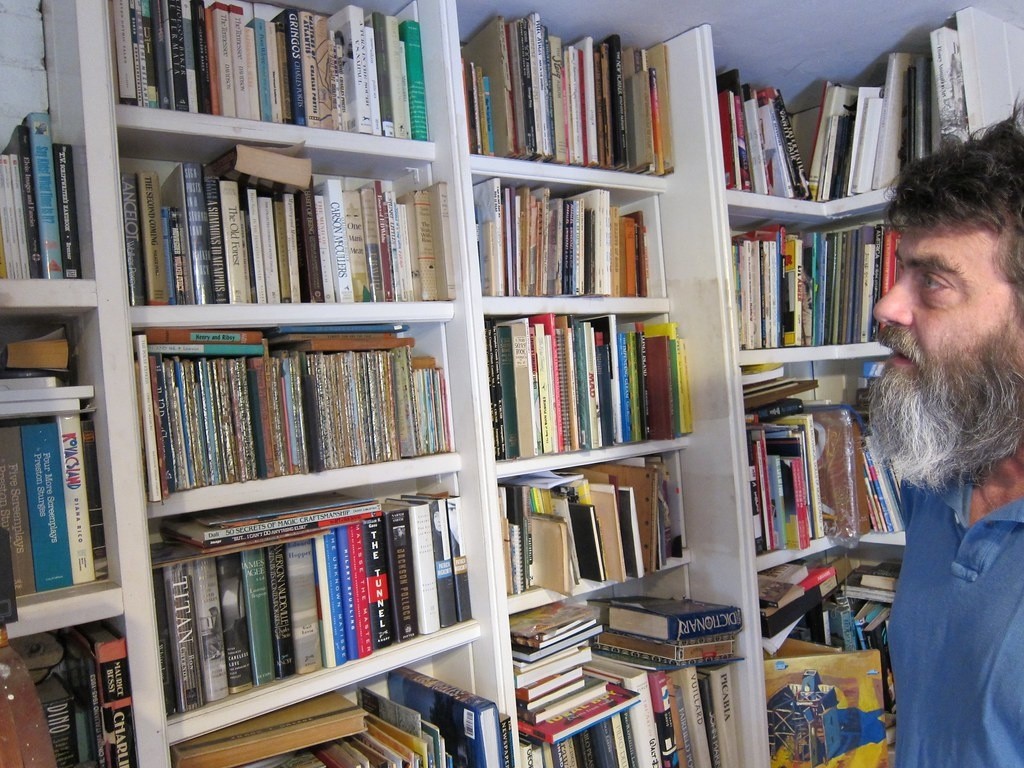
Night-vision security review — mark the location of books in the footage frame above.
[0,0,1024,768]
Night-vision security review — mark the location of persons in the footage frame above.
[867,96,1024,768]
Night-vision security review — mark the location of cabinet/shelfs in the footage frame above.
[0,0,1024,768]
